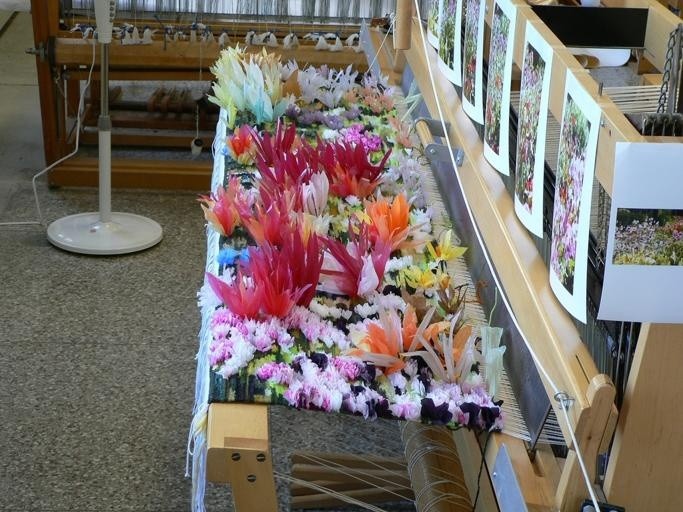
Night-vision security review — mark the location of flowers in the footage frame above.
[199,47,509,434]
[426,0,683,296]
